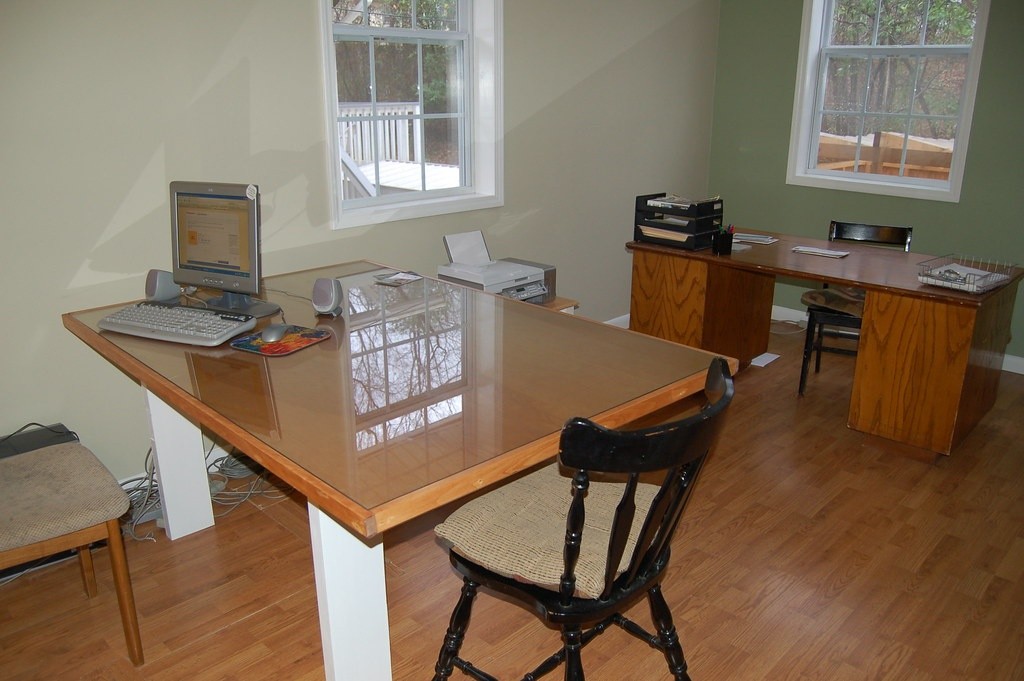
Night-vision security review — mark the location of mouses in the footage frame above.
[263,323,295,343]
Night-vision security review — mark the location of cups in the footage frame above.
[712,230,732,255]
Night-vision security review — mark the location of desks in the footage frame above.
[625,222,1024,467]
[63,258,738,681]
[535,296,578,319]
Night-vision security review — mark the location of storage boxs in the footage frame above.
[633,225,721,251]
[634,193,724,219]
[635,213,723,233]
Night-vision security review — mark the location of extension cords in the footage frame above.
[130,481,227,525]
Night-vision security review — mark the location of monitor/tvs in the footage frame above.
[170,181,281,320]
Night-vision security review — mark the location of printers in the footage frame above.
[437,230,557,305]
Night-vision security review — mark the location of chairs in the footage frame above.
[433,357,735,681]
[796,220,914,399]
[0,442,145,668]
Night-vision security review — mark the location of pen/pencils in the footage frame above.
[718,223,736,234]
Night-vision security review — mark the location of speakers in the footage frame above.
[311,278,344,317]
[145,269,181,306]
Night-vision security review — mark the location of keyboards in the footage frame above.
[98,304,257,347]
